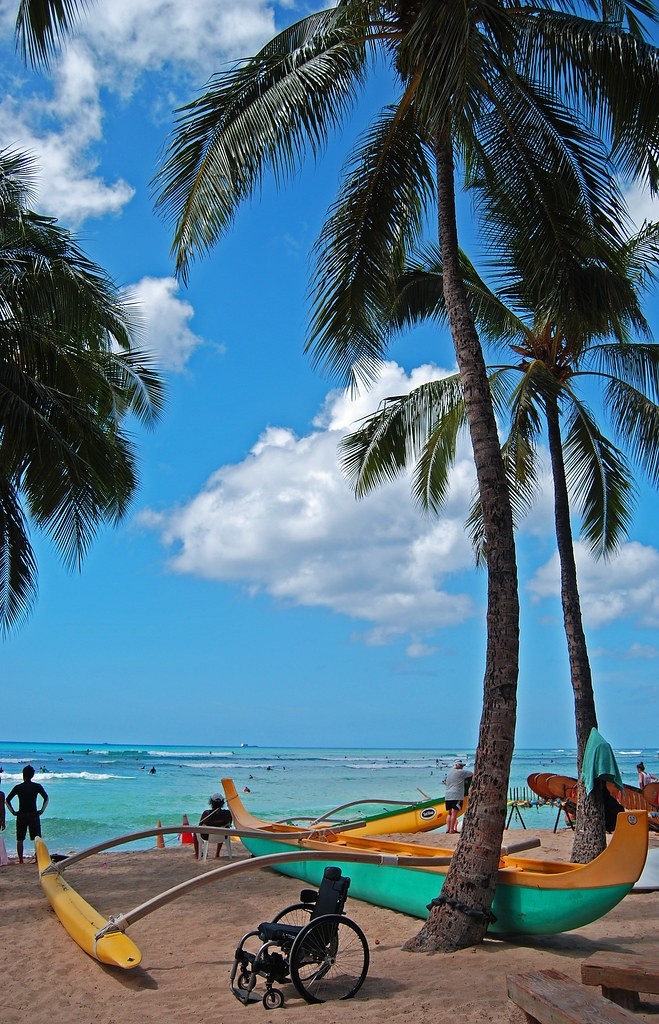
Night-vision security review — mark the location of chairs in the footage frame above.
[195,822,233,860]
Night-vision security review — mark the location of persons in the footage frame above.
[636,761,658,788]
[188,793,232,860]
[444,759,473,834]
[150,767,156,774]
[0,765,49,866]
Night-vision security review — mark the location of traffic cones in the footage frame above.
[181,814,194,844]
[157,820,166,849]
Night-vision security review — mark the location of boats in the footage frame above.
[232,776,472,842]
[221,775,650,942]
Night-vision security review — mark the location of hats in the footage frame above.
[212,793,223,802]
[453,759,466,766]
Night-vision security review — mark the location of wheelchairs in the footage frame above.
[227,866,370,1010]
